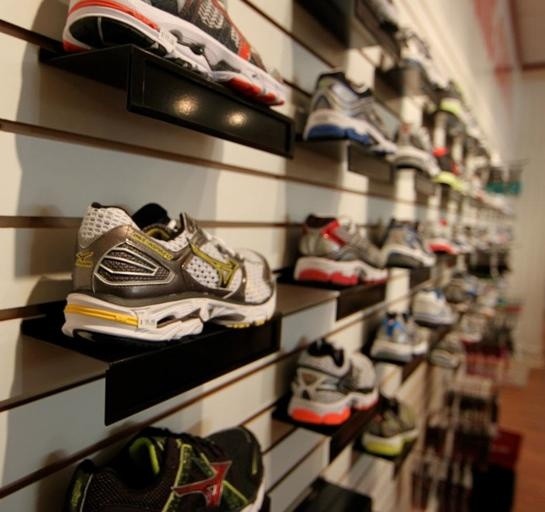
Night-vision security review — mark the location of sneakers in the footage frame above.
[288,337,383,427]
[294,210,390,289]
[54,196,281,343]
[58,420,269,512]
[302,68,401,159]
[60,0,292,106]
[360,394,420,459]
[361,0,402,33]
[368,28,517,371]
